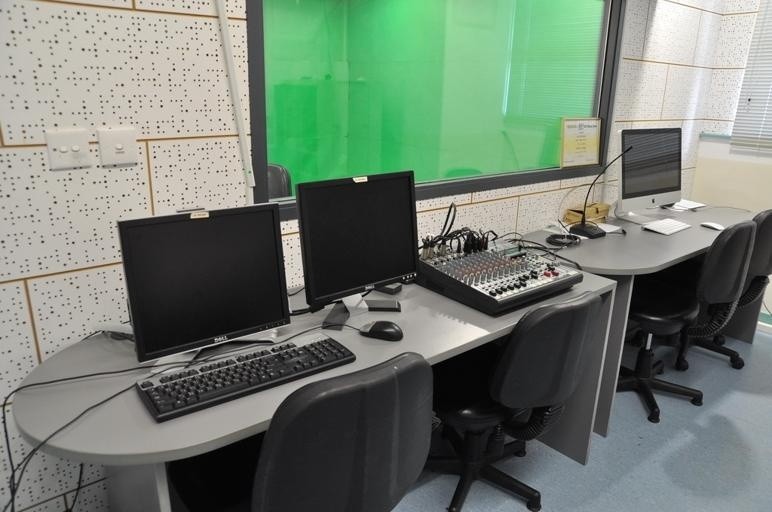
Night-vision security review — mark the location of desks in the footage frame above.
[11,240,619,512]
[515,202,760,438]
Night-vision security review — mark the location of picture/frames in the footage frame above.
[560,117,602,169]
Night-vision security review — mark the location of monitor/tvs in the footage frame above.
[117,202,291,374]
[614,128,683,225]
[295,170,420,330]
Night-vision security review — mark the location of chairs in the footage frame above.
[631,209,772,371]
[170,352,433,512]
[616,220,757,423]
[424,291,601,512]
[267,163,292,201]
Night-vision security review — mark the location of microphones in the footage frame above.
[569,145,633,239]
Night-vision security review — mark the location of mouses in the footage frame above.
[700,223,725,231]
[359,320,403,342]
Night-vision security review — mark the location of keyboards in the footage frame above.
[135,331,357,425]
[641,218,692,236]
